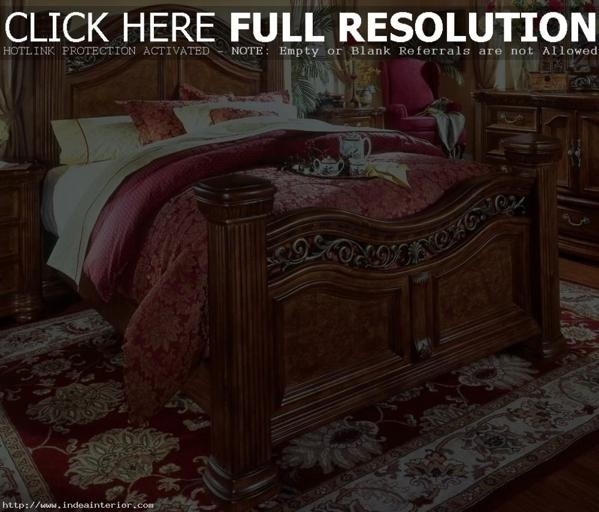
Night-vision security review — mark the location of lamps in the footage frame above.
[344,23,365,109]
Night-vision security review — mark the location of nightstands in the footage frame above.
[0,154,49,327]
[316,107,387,128]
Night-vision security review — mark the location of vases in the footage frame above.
[360,84,374,105]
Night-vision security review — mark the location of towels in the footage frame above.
[367,158,415,190]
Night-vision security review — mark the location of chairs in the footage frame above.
[382,51,469,160]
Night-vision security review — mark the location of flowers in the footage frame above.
[359,64,381,86]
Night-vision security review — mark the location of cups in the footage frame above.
[312,156,345,177]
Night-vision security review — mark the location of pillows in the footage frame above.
[49,81,299,164]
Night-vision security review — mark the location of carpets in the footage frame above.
[1,279,597,509]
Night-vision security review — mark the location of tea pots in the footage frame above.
[339,131,373,160]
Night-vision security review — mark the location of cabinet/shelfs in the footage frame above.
[475,89,599,267]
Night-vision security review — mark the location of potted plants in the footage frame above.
[501,0,587,91]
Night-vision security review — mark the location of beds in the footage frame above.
[32,5,567,508]
